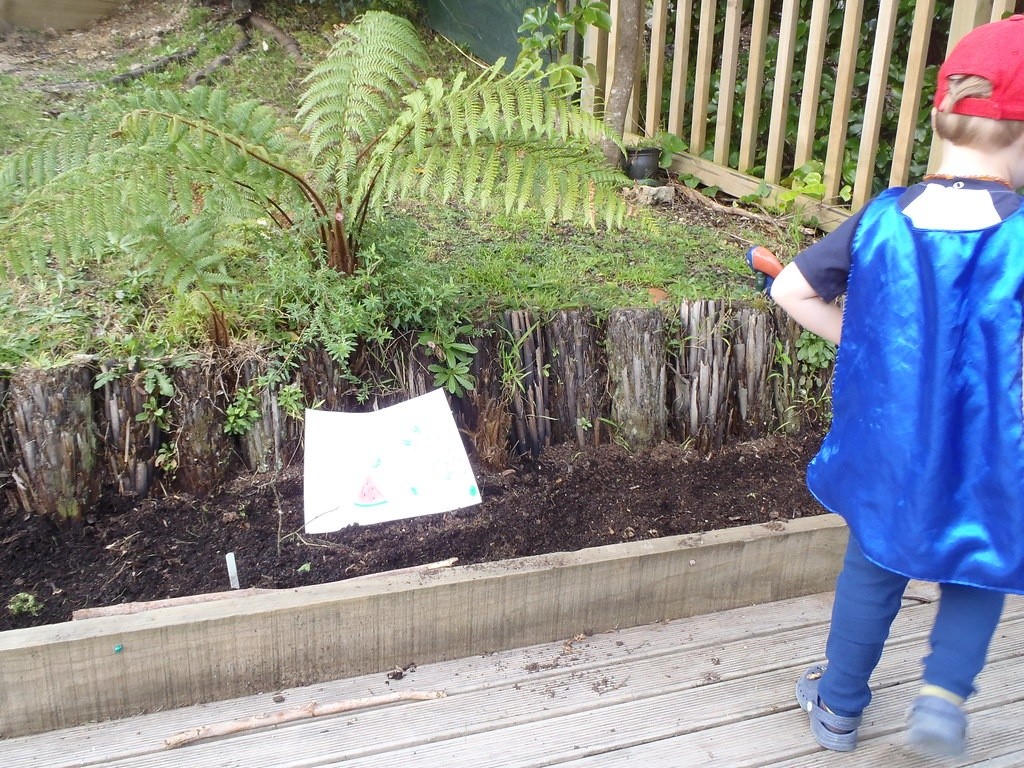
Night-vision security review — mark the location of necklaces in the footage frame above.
[923,172,1015,193]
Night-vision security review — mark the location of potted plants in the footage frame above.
[625,141,662,180]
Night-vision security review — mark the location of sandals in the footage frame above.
[903,696,968,756]
[796,664,863,751]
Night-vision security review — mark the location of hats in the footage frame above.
[934,14,1024,119]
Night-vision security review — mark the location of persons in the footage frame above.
[771,14,1024,752]
